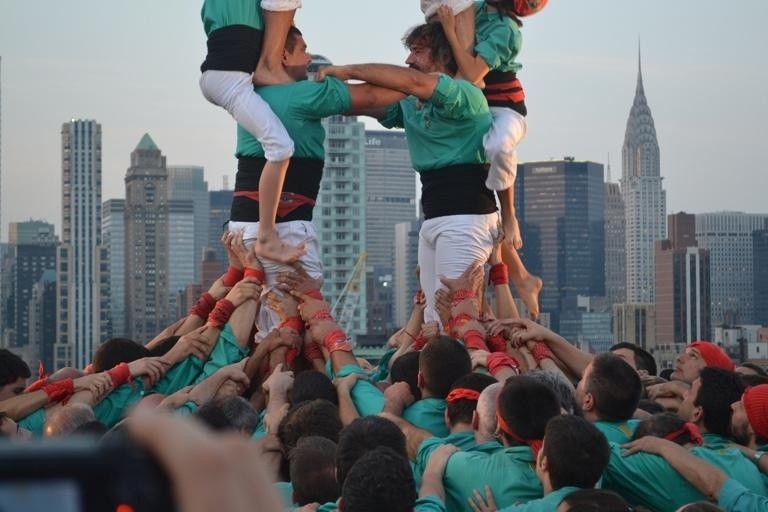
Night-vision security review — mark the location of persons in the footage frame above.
[250,1,302,85]
[435,1,547,321]
[199,1,307,267]
[312,22,503,340]
[224,22,411,357]
[420,1,485,90]
[1,220,766,511]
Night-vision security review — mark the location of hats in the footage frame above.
[513,0,547,16]
[744,383,768,437]
